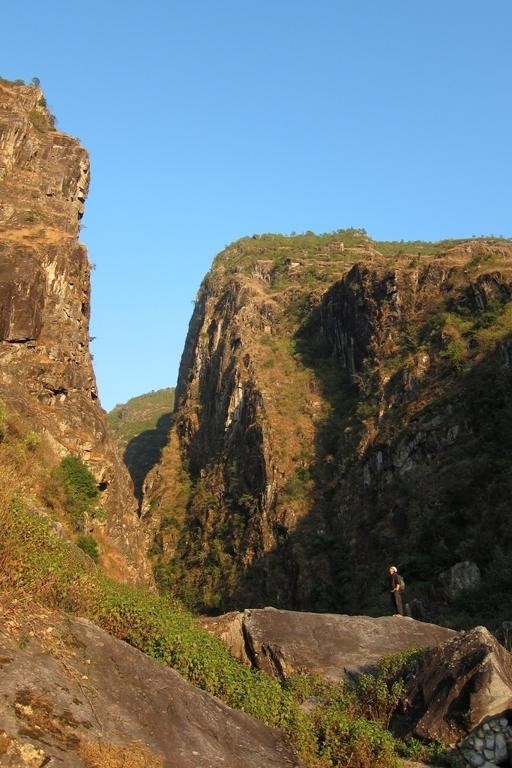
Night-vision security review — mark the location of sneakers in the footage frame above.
[396,614,402,616]
[393,614,396,616]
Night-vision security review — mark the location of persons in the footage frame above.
[388,565,406,615]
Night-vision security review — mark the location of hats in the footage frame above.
[391,567,397,572]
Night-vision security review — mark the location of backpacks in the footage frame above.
[396,575,405,590]
